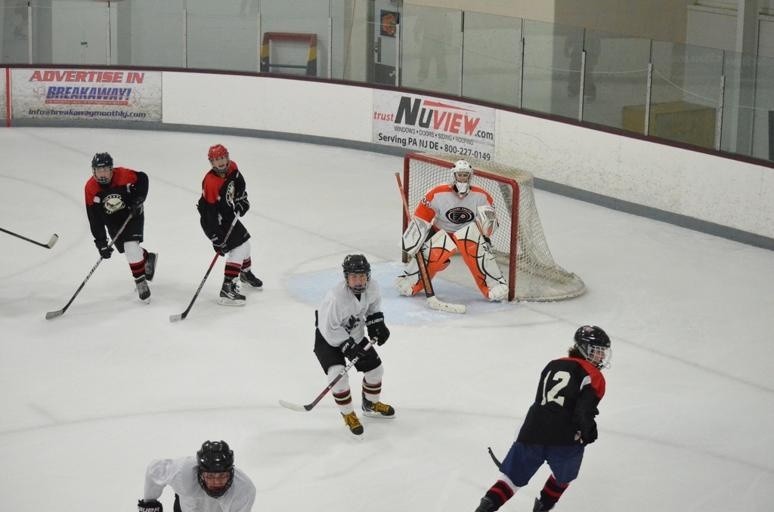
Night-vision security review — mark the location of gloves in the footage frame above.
[365,313,390,346]
[209,232,229,256]
[339,338,367,362]
[233,191,250,216]
[94,239,113,258]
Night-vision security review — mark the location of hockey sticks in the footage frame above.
[488,446,504,469]
[394,172,467,315]
[45,211,133,322]
[169,210,241,323]
[277,337,377,413]
[0,227,59,249]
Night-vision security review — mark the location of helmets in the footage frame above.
[575,325,611,372]
[92,151,113,184]
[342,255,371,294]
[195,441,234,498]
[452,159,471,197]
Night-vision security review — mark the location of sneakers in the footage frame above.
[219,277,246,300]
[240,266,262,288]
[341,409,363,434]
[363,393,394,415]
[135,252,155,300]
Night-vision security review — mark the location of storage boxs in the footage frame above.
[621,100,716,142]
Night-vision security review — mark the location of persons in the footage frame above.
[313,251,397,436]
[396,159,506,304]
[416,8,455,82]
[195,142,266,303]
[140,437,257,512]
[472,324,614,512]
[84,150,157,302]
[564,29,599,99]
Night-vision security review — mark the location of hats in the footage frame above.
[208,144,228,159]
